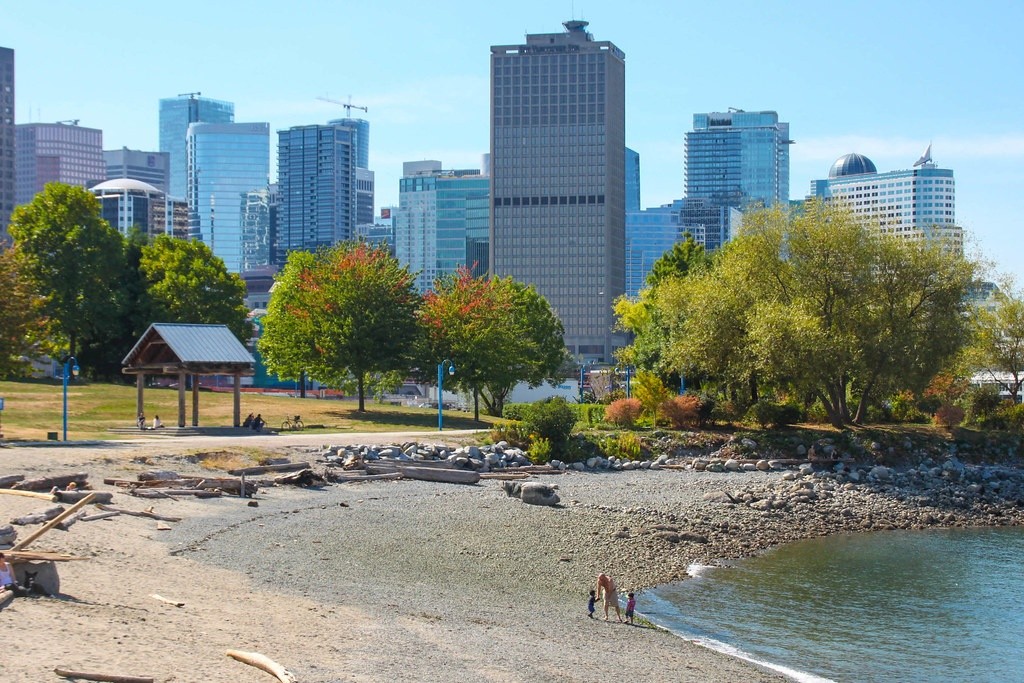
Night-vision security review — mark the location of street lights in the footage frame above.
[438,358,456,431]
[63,356,82,441]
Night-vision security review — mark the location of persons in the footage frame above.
[623,593,635,625]
[808,444,818,462]
[244,414,254,426]
[831,450,841,459]
[0,554,26,596]
[298,470,323,487]
[597,573,622,622]
[138,412,146,430]
[51,483,77,493]
[253,414,267,432]
[152,415,159,429]
[587,590,600,619]
